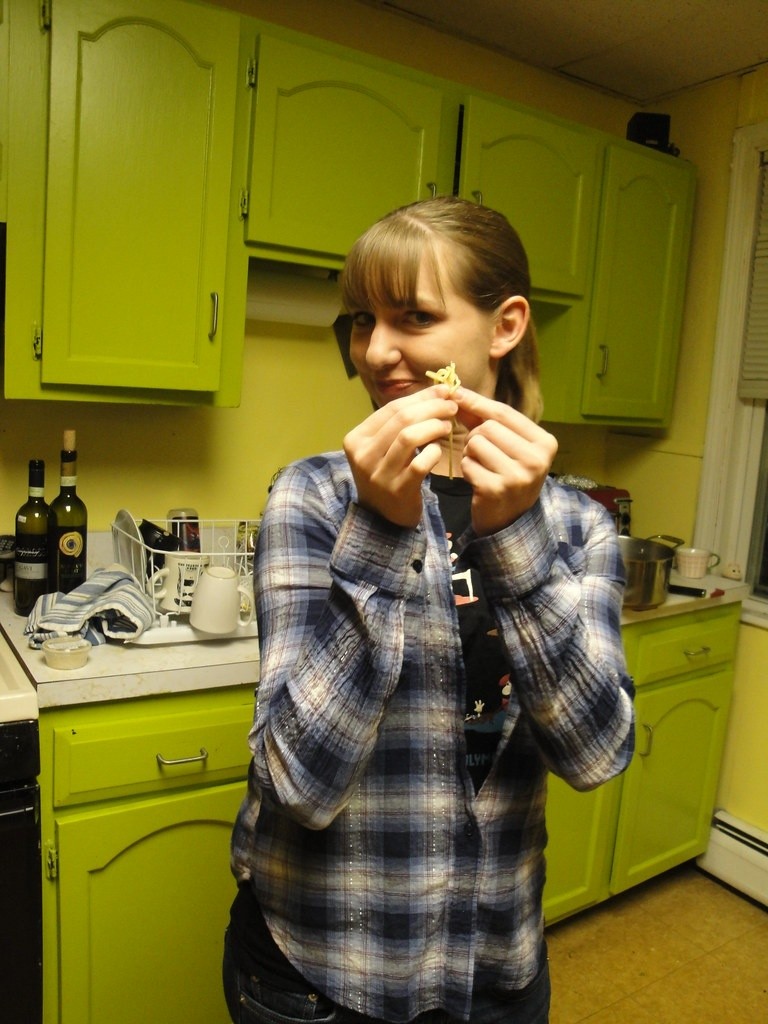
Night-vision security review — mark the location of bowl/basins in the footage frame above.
[42,637,91,670]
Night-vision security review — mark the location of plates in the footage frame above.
[115,510,147,584]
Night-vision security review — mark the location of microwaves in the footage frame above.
[582,485,631,537]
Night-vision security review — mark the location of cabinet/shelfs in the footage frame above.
[241,18,601,307]
[35,688,255,1024]
[0,0,248,408]
[540,600,746,931]
[530,133,700,432]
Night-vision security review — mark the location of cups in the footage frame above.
[188,566,255,634]
[147,553,210,614]
[675,548,721,579]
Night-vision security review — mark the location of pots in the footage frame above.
[614,535,685,608]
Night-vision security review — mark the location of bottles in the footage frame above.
[50,430,87,594]
[15,460,51,618]
[167,508,201,552]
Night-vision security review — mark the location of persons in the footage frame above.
[222,196,635,1024]
